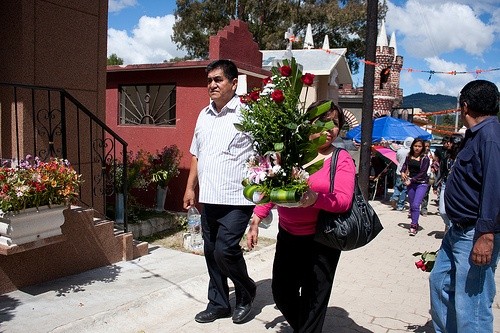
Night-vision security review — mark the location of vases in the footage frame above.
[0,202,69,248]
[114,192,125,226]
[158,183,168,212]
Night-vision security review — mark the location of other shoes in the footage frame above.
[397,206,406,210]
[408,222,418,235]
[390,199,397,210]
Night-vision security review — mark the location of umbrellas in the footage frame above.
[369,144,406,186]
[344,114,433,150]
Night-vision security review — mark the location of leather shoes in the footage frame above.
[232,291,256,323]
[194,309,230,323]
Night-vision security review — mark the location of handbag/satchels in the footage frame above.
[316,147,384,252]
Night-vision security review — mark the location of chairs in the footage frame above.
[370,167,392,201]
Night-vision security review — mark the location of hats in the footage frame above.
[440,136,450,144]
[403,137,414,149]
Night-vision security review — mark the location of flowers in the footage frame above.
[413,250,439,274]
[0,155,80,206]
[233,57,334,203]
[102,140,183,191]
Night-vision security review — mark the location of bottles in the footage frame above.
[188,206,203,250]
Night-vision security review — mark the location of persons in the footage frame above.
[182,58,272,323]
[423,80,500,333]
[368,131,466,238]
[244,98,355,333]
